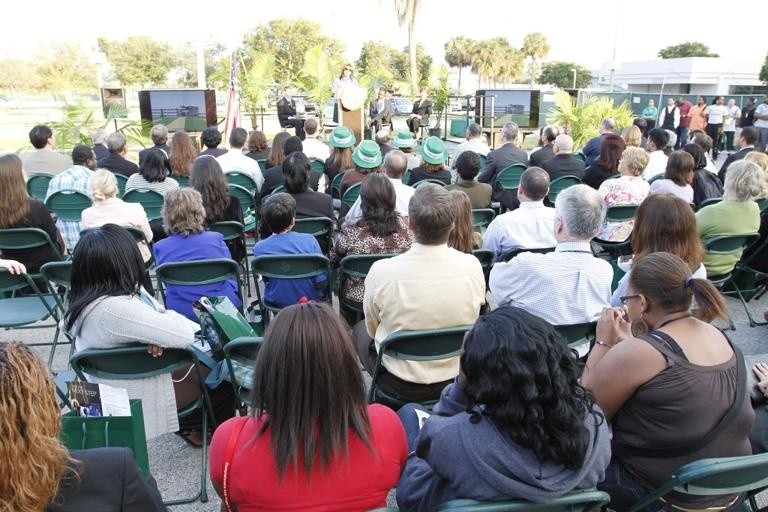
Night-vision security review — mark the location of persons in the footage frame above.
[3,116,768,445]
[331,64,361,123]
[385,305,618,512]
[2,336,176,511]
[206,299,410,512]
[367,87,394,135]
[581,253,761,511]
[277,85,307,141]
[641,94,768,162]
[405,86,434,139]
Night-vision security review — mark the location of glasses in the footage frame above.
[621,296,641,304]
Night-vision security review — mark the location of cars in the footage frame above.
[215,90,475,132]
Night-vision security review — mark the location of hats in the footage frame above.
[392,131,417,148]
[419,136,449,164]
[329,125,356,147]
[351,139,382,169]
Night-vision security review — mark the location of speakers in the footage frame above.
[100,86,127,118]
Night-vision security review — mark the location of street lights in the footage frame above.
[570,69,578,90]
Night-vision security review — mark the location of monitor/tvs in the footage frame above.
[138,90,217,136]
[475,90,540,133]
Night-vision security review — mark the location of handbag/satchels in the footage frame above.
[59,399,149,484]
[193,295,261,361]
